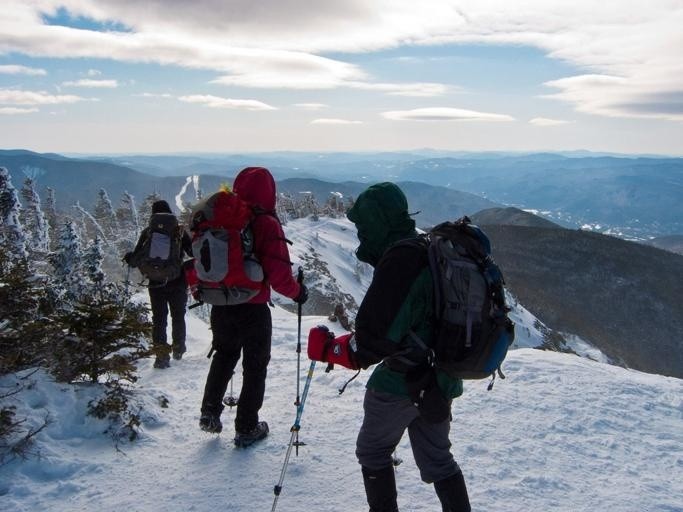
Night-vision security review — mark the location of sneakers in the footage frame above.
[234,421,269,448]
[198,414,222,434]
[153,346,186,369]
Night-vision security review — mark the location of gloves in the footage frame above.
[308,327,360,370]
[293,282,310,304]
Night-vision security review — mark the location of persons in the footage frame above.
[124,200,196,368]
[305,178,472,512]
[177,166,309,448]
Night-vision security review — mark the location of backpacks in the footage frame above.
[140,213,180,281]
[189,191,281,306]
[374,216,514,380]
